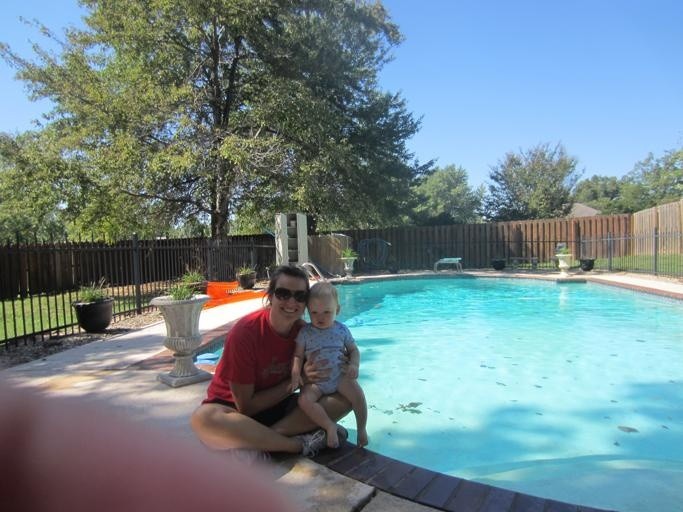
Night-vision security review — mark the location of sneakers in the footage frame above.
[233,448,271,466]
[299,424,348,456]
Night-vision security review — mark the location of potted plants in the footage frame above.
[235,263,258,289]
[555,245,572,275]
[70,276,113,333]
[336,247,358,279]
[151,266,212,386]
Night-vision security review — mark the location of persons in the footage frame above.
[190,263,355,464]
[285,281,368,450]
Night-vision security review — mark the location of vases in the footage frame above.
[491,258,506,270]
[579,256,595,271]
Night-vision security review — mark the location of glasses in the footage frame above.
[275,288,307,302]
